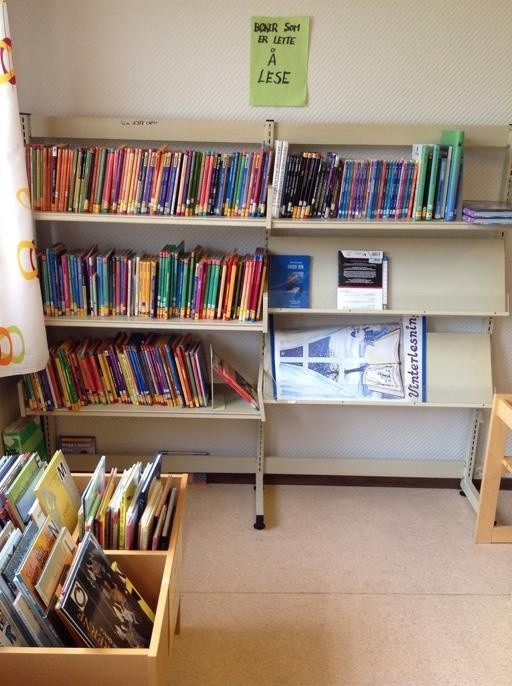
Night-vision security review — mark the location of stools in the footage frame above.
[472,389,511,548]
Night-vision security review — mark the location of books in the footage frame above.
[2,415,50,461]
[0,449,176,650]
[157,447,210,457]
[17,330,260,409]
[59,434,96,456]
[165,469,208,487]
[269,252,312,309]
[267,130,510,224]
[26,145,275,218]
[339,250,387,311]
[37,241,271,321]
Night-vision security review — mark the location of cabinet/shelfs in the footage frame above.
[1,465,191,686]
[14,112,271,531]
[256,116,511,529]
